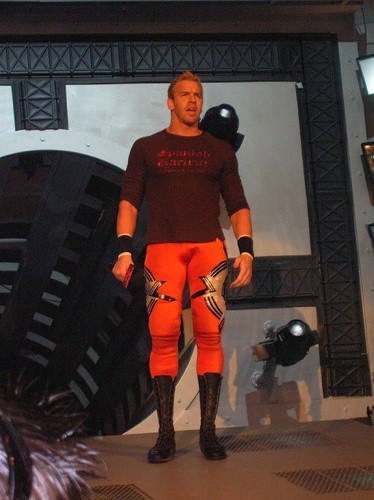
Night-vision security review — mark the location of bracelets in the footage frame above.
[237,234,255,260]
[116,233,134,258]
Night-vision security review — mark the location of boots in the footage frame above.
[147,375,177,463]
[197,372,226,460]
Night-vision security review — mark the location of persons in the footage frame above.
[0,386,101,500]
[108,69,254,463]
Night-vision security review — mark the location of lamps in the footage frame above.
[356,53,374,96]
[361,136,374,206]
[251,320,317,389]
[198,104,245,154]
